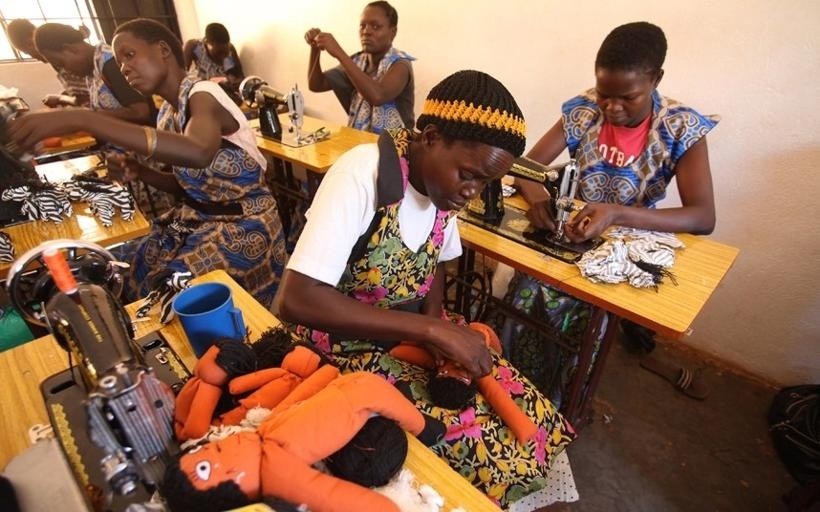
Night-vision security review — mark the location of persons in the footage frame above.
[274,70,581,512]
[5,20,288,311]
[157,324,447,511]
[6,18,90,109]
[388,321,538,445]
[184,23,246,107]
[158,326,447,512]
[33,22,157,162]
[304,0,416,138]
[488,21,724,422]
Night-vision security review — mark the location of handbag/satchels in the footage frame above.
[771,384,820,488]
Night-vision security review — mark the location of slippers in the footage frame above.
[641,355,710,399]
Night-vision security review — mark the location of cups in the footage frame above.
[170,283,247,359]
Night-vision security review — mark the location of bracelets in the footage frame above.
[144,125,157,162]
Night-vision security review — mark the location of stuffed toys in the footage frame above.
[135,267,193,323]
[0,174,136,264]
